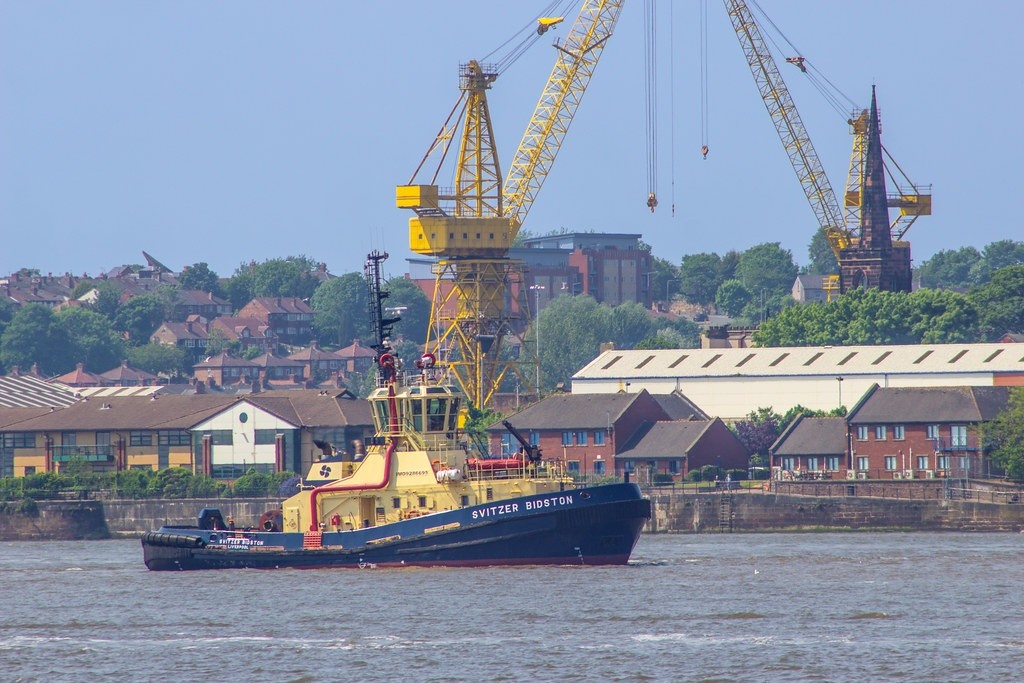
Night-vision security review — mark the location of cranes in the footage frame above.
[720,0,935,299]
[391,0,630,460]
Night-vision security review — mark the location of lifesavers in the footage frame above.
[431,460,444,472]
[408,510,420,519]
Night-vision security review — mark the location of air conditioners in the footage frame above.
[857,473,867,480]
[905,470,914,479]
[892,473,902,479]
[846,469,855,479]
[926,470,934,478]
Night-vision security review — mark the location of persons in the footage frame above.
[715,474,732,492]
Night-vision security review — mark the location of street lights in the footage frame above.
[115,431,123,472]
[835,376,844,409]
[845,432,854,471]
[42,433,50,472]
[529,283,545,393]
[184,430,193,473]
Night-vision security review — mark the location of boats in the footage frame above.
[466,451,531,471]
[137,246,655,570]
